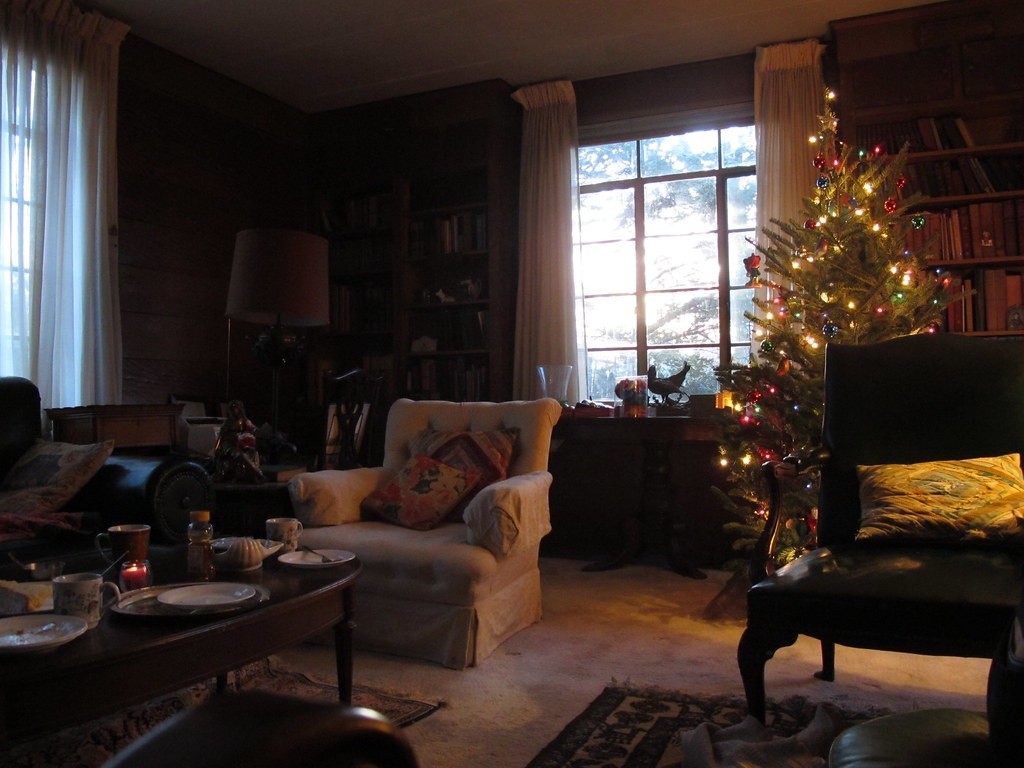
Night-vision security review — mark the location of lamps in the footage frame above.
[225,228,330,455]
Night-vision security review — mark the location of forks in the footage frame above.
[302,544,343,563]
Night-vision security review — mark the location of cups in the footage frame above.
[613,376,648,417]
[265,518,303,556]
[536,365,572,414]
[95,524,151,574]
[119,559,153,592]
[52,572,121,631]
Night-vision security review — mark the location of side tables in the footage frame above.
[211,476,291,539]
[47,402,186,456]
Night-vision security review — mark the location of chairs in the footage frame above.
[829,706,1023,766]
[736,335,1024,724]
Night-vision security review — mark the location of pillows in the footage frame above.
[2,435,117,515]
[406,429,521,520]
[359,455,480,529]
[854,451,1024,548]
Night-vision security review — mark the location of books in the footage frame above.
[856,117,975,152]
[308,188,486,408]
[857,266,1024,342]
[905,200,1024,260]
[886,155,997,197]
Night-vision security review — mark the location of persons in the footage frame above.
[214,400,272,484]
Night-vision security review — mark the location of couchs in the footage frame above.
[0,379,212,567]
[291,399,564,669]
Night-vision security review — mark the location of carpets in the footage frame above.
[0,656,441,767]
[526,688,881,768]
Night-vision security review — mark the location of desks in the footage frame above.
[567,417,727,579]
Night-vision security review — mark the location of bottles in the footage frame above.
[186,511,217,583]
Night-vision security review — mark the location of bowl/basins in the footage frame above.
[14,560,66,583]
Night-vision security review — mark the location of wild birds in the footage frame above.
[646,361,691,404]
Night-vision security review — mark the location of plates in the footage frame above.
[0,615,88,654]
[158,583,256,610]
[278,549,356,569]
[17,581,54,612]
[213,540,284,558]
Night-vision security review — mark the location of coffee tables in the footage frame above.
[0,538,363,746]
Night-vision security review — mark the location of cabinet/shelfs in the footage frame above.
[829,0,1024,334]
[302,159,399,440]
[400,172,518,406]
